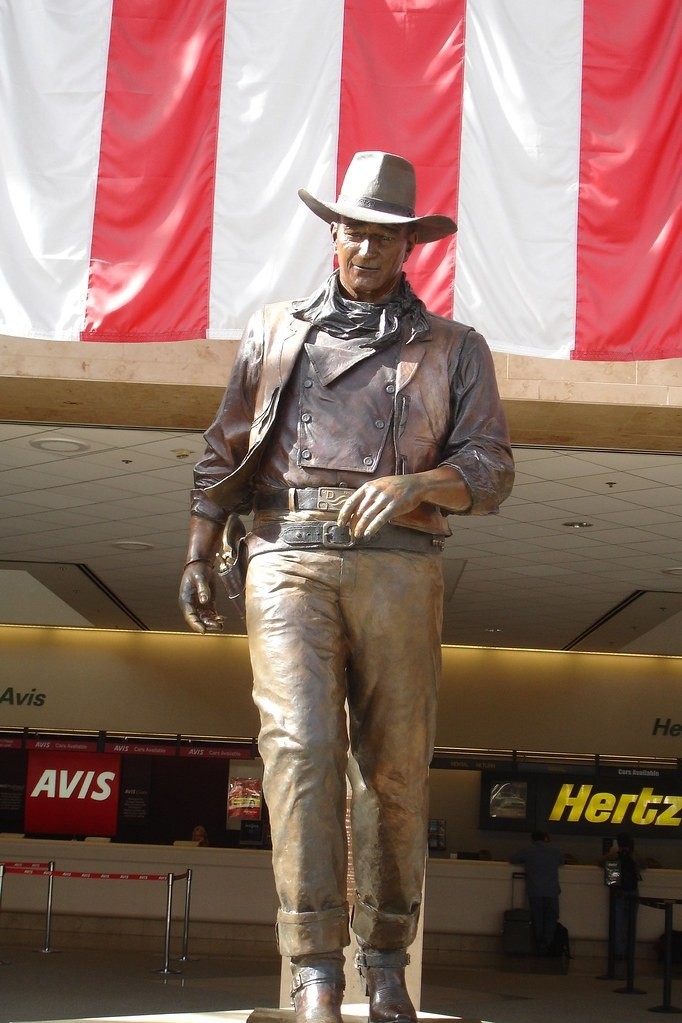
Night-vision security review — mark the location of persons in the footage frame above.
[192,825,209,847]
[509,830,568,957]
[178,152,517,1023]
[598,831,642,962]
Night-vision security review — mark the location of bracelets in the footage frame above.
[182,558,216,571]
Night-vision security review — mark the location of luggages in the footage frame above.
[504,872,533,958]
